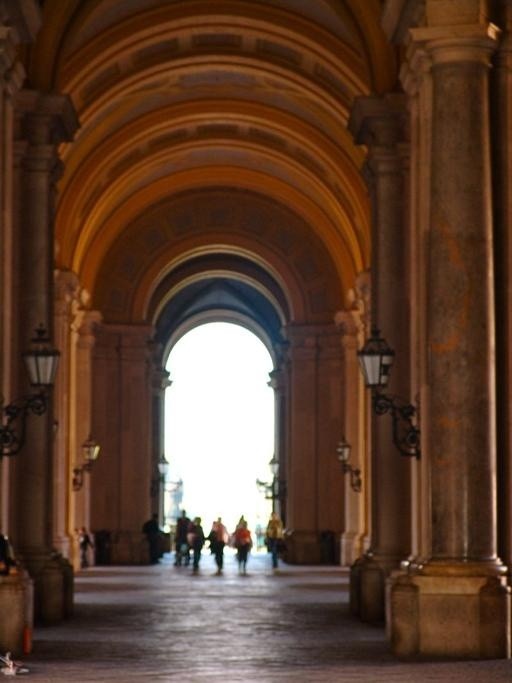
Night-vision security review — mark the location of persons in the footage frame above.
[74,527,96,570]
[165,509,263,577]
[266,511,286,572]
[0,532,19,577]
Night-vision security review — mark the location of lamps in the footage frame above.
[73,434,101,491]
[0,321,61,459]
[149,454,169,495]
[353,327,421,460]
[267,454,286,493]
[335,436,361,491]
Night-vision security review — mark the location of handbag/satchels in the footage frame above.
[278,540,287,552]
[187,532,200,546]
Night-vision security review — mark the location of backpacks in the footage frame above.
[143,521,154,534]
